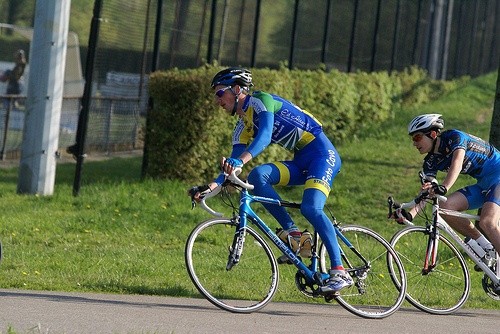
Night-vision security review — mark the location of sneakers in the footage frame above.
[278,236,300,264]
[319,269,353,296]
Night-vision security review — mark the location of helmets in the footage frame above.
[211,67,252,96]
[408,113,444,140]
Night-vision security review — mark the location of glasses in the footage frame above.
[413,132,430,142]
[216,86,231,97]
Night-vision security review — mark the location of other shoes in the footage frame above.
[474,254,492,272]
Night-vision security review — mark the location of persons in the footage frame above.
[388,114,500,272]
[188,67,354,292]
[6,50,26,94]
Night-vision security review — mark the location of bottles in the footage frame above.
[464,237,486,259]
[300,229,311,258]
[275,227,299,253]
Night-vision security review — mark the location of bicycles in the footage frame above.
[183,162,407,319]
[387,170,500,315]
